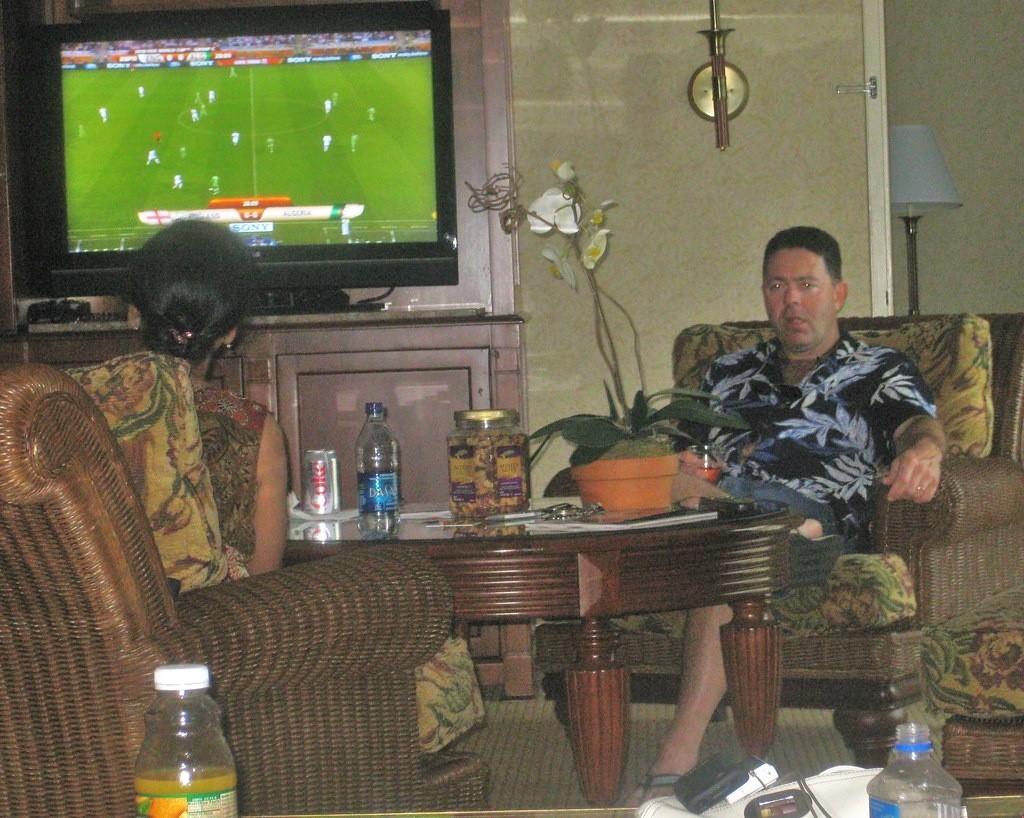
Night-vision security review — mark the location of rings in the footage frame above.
[917,487,924,490]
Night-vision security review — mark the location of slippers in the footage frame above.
[635,773,683,808]
[774,526,846,602]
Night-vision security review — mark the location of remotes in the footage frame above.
[698,496,754,514]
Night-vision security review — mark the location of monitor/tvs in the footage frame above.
[29,9,458,317]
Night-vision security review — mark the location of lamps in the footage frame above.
[888,124,966,315]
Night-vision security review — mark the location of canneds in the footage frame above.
[303,448,341,513]
[446,408,530,518]
[303,519,340,542]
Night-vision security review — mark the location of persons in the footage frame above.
[62,30,432,195]
[123,214,288,582]
[623,226,946,807]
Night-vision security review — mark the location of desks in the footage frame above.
[282,505,809,818]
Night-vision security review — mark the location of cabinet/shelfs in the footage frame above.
[0,312,537,700]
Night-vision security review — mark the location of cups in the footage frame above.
[680,444,722,487]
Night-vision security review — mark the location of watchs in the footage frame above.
[545,501,606,520]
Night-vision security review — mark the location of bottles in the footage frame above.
[131,664,236,816]
[356,402,399,539]
[867,723,962,817]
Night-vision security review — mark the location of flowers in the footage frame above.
[522,161,748,485]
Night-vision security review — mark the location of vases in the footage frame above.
[565,451,676,516]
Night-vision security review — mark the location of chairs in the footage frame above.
[0,364,490,818]
[535,312,1024,818]
